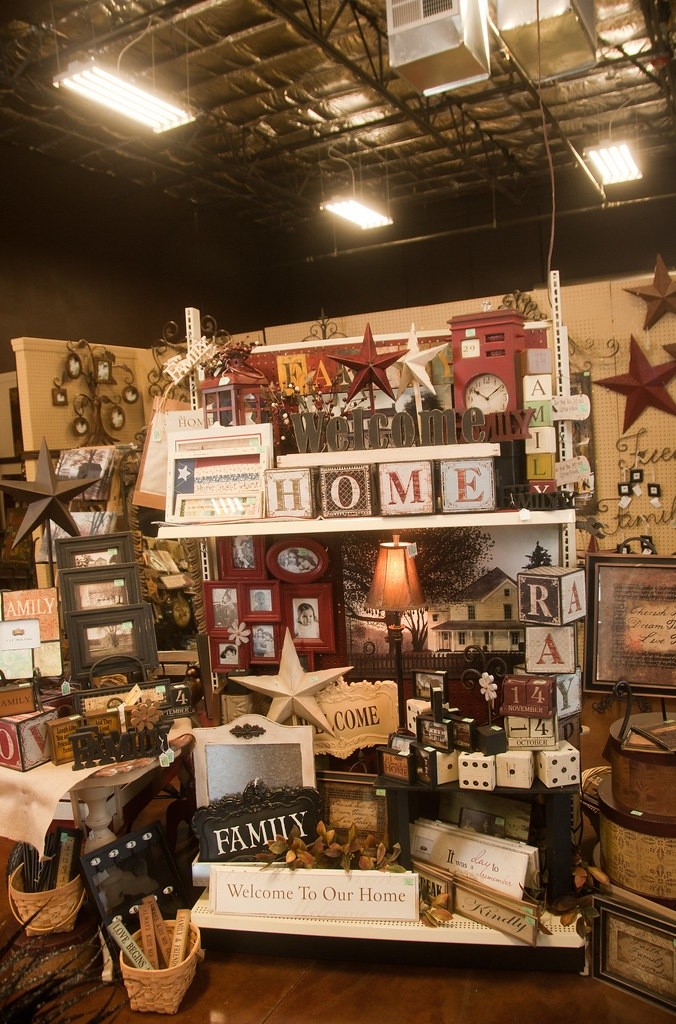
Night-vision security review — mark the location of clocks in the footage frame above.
[462,373,510,413]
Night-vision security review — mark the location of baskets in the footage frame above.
[119,920,204,1015]
[11,865,87,936]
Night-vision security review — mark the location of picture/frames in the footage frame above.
[174,491,265,523]
[281,583,334,652]
[55,530,135,570]
[582,551,676,699]
[238,578,282,622]
[58,562,145,612]
[163,444,269,522]
[241,622,281,667]
[212,635,248,674]
[206,582,240,636]
[63,603,157,676]
[219,534,266,580]
[167,423,275,469]
[266,539,329,582]
[51,338,139,436]
[591,893,676,1014]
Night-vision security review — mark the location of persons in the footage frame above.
[250,590,270,610]
[254,628,275,657]
[295,602,319,638]
[285,551,314,572]
[216,592,236,626]
[220,644,237,664]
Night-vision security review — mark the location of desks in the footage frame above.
[0,747,182,853]
[392,773,583,915]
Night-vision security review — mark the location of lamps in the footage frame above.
[45,11,196,134]
[364,533,426,745]
[585,92,642,186]
[320,131,394,230]
